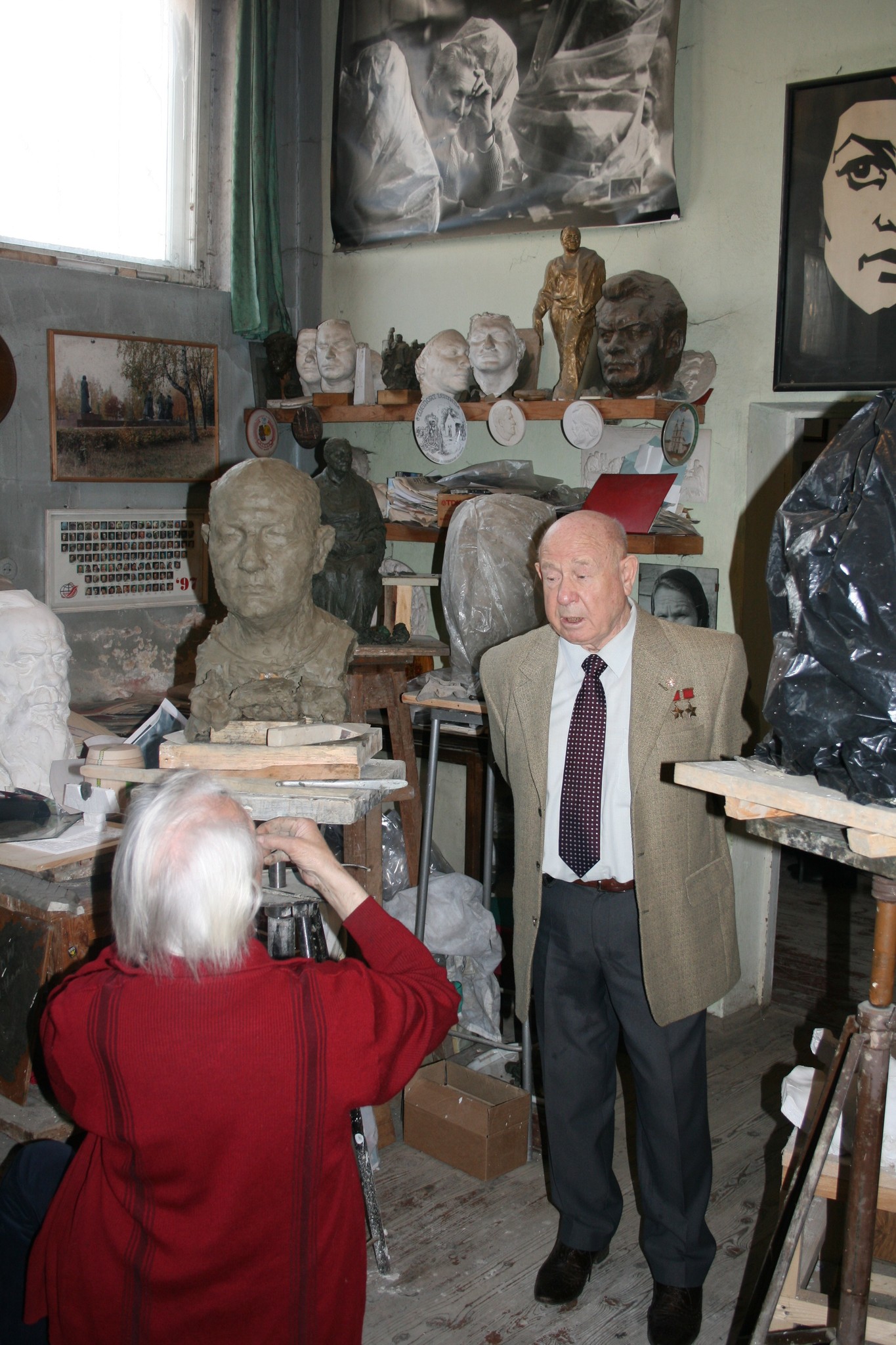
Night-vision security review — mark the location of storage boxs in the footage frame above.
[404,1059,529,1182]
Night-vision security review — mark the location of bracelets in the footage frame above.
[474,121,496,141]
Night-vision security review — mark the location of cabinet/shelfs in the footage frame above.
[267,395,705,559]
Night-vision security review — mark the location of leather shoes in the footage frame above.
[532,1241,611,1306]
[646,1284,703,1345]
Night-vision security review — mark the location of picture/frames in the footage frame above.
[46,328,219,485]
[44,508,208,614]
[773,62,896,387]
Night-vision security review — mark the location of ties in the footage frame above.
[559,654,609,879]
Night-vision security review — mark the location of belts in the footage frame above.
[573,877,635,893]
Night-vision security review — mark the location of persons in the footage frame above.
[26,773,457,1345]
[80,375,93,413]
[649,567,710,630]
[0,588,78,792]
[416,329,473,403]
[419,42,505,218]
[143,389,174,421]
[310,438,388,638]
[187,457,359,741]
[532,225,606,400]
[594,269,688,400]
[380,327,426,390]
[479,512,755,1344]
[296,319,359,394]
[467,311,525,402]
[61,519,195,596]
